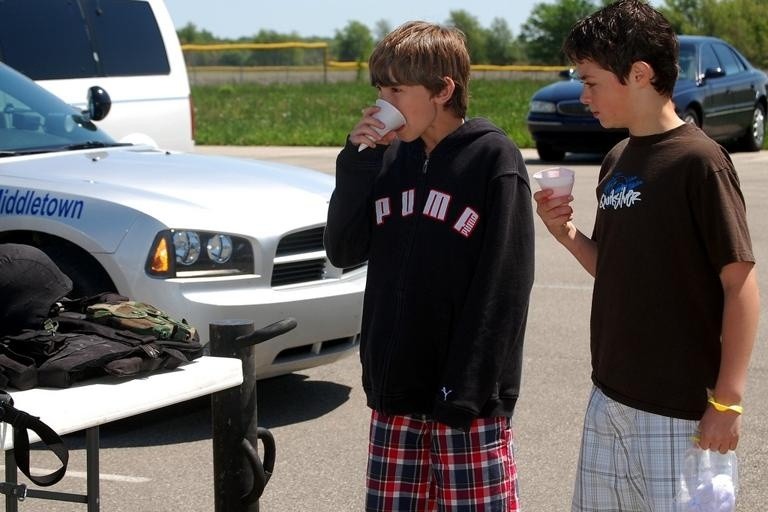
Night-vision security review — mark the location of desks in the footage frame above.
[0,354,244,512]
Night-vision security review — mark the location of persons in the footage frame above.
[532,1,761,512]
[323,20,536,512]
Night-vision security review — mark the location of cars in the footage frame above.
[0,62,370,378]
[523,34,766,156]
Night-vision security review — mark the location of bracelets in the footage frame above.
[706,388,744,415]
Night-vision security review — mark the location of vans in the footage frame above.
[1,1,198,152]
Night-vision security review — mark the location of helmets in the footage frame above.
[0,242,73,327]
